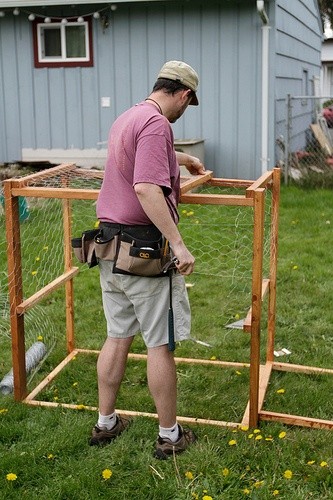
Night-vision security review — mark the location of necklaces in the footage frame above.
[145,98,163,116]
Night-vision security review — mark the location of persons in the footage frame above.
[86,60,209,459]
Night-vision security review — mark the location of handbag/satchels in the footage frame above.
[71,225,174,278]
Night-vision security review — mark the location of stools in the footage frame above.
[174,138,207,193]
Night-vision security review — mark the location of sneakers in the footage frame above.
[92,417,132,445]
[153,424,197,461]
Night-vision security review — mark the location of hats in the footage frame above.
[157,60,199,106]
